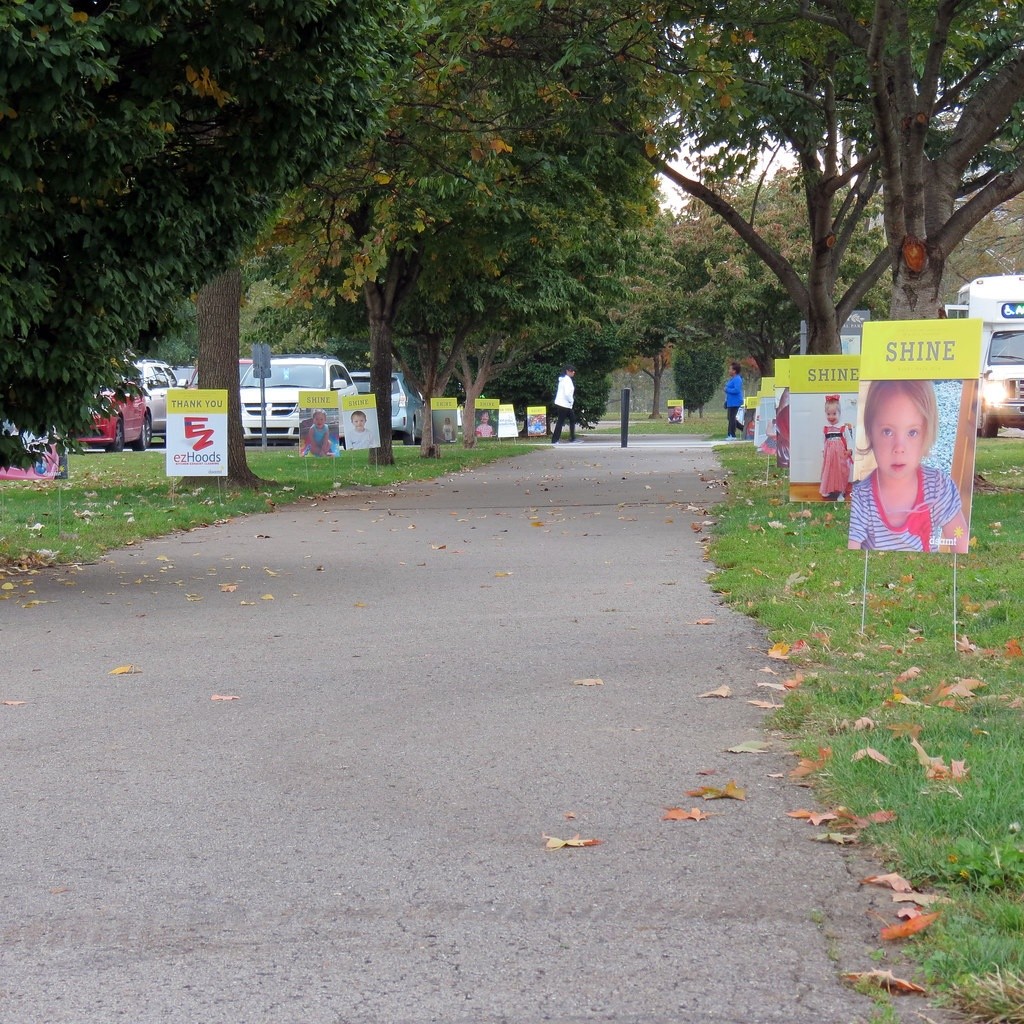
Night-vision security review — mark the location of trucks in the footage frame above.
[945,275,1024,437]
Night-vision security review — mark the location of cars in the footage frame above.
[188,358,251,392]
[349,372,425,446]
[170,366,195,387]
[69,382,153,453]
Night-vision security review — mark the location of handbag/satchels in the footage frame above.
[723,402,727,409]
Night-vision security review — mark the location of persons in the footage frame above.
[819,395,853,502]
[668,406,682,423]
[442,418,453,442]
[475,410,493,437]
[309,409,332,457]
[723,362,744,440]
[551,365,584,445]
[345,411,375,450]
[745,410,755,440]
[849,380,968,553]
[760,418,777,454]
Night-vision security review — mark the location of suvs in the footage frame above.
[240,356,358,447]
[123,358,178,446]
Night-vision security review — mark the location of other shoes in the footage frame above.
[552,441,559,444]
[822,492,831,497]
[725,435,737,441]
[570,439,585,444]
[836,496,845,502]
